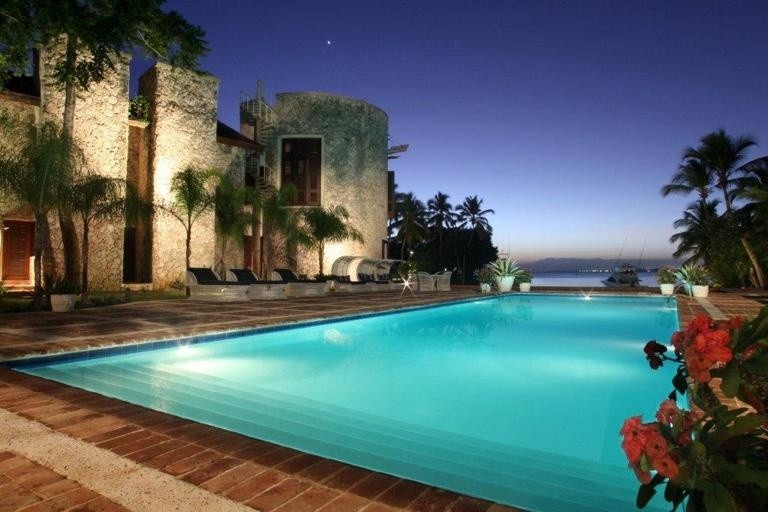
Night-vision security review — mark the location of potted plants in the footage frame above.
[49,275,81,313]
[473,255,530,294]
[657,263,710,297]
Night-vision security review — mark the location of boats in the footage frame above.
[599,270,639,287]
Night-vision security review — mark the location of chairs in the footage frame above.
[187,267,453,302]
